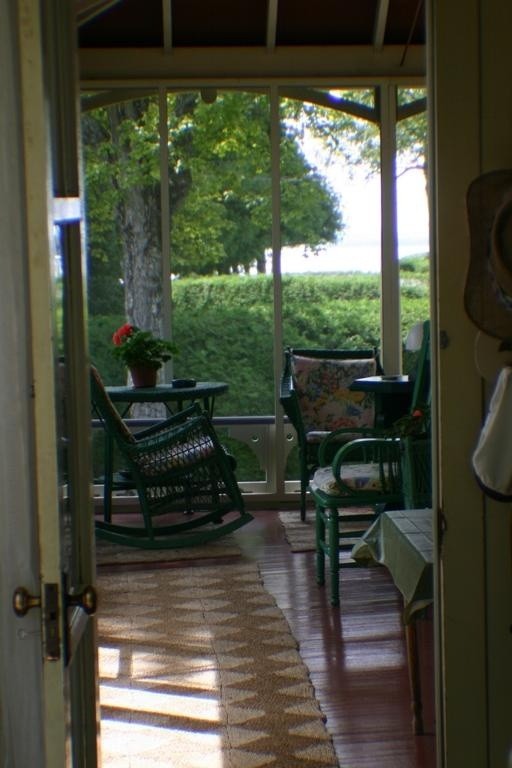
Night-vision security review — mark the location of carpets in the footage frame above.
[83,519,341,766]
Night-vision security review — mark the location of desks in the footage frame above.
[98,378,232,523]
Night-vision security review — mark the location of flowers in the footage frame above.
[111,323,177,361]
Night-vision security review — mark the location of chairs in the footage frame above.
[88,363,255,553]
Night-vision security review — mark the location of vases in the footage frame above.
[124,356,161,387]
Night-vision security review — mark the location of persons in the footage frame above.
[463,167,512,502]
